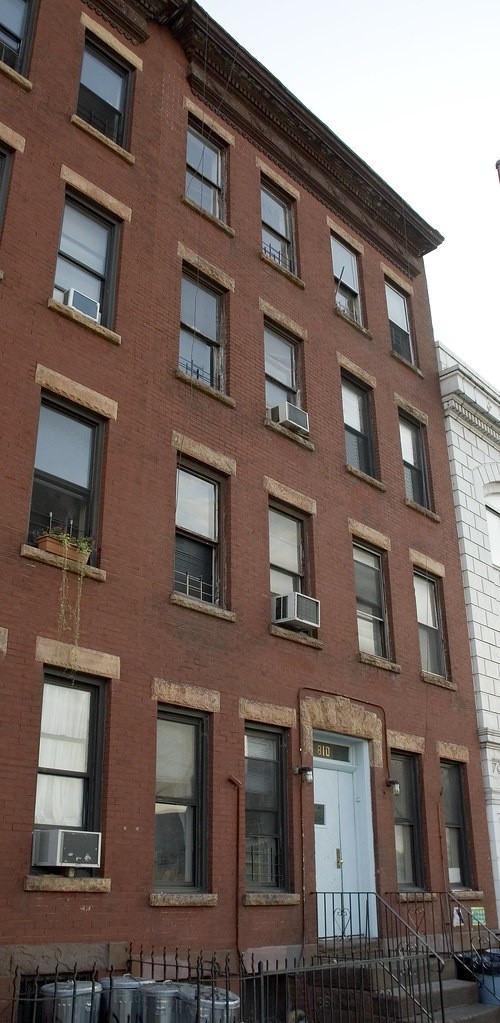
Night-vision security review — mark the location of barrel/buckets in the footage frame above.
[175,984,240,1023]
[41,981,102,1023]
[138,980,189,1023]
[98,974,156,1023]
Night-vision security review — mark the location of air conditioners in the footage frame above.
[31,828,101,868]
[63,287,101,322]
[268,401,309,434]
[271,591,320,630]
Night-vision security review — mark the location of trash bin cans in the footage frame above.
[175,983,241,1023]
[140,979,186,1023]
[100,974,156,1023]
[455,948,500,1005]
[39,978,103,1023]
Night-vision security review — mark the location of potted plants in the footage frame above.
[35,528,92,686]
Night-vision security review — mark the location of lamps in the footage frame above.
[298,767,313,784]
[385,779,400,795]
